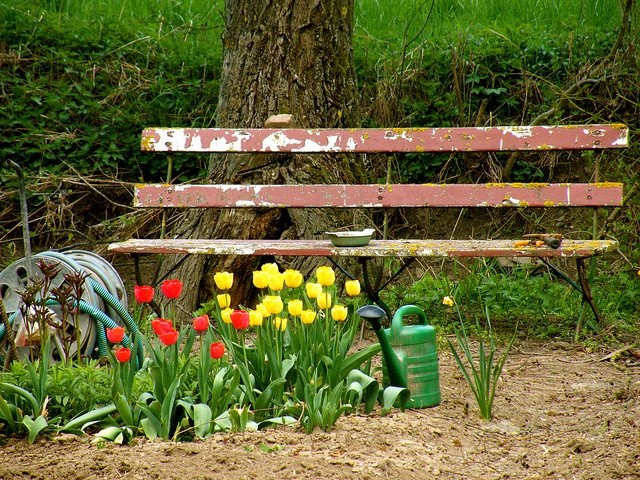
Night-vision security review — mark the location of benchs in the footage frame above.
[106,122,629,322]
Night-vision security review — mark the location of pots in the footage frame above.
[325,227,376,246]
